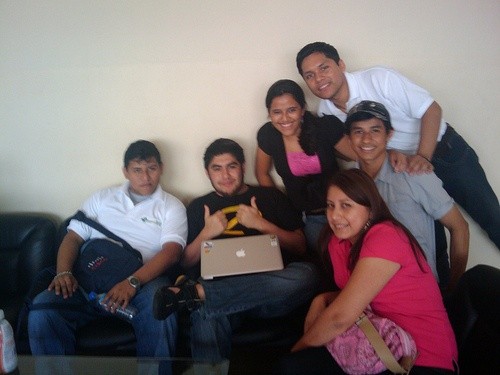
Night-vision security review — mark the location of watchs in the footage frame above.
[127,275,140,290]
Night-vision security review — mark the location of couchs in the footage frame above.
[0,210,303,361]
[444,264,500,375]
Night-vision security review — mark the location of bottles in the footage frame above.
[88,291,139,321]
[0,309,17,374]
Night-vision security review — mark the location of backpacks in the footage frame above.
[56,211,143,295]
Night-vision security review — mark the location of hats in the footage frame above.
[347,100,390,121]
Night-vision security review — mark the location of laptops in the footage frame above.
[200,235,284,279]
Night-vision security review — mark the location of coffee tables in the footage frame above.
[7,352,231,375]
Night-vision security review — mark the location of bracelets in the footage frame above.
[53,271,72,279]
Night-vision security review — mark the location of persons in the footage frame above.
[28,140,188,356]
[152,138,319,358]
[297,42,500,273]
[255,80,407,272]
[270,168,458,375]
[340,100,471,307]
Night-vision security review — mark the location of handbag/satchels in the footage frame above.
[324,304,416,375]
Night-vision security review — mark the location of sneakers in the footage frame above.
[152,275,200,320]
